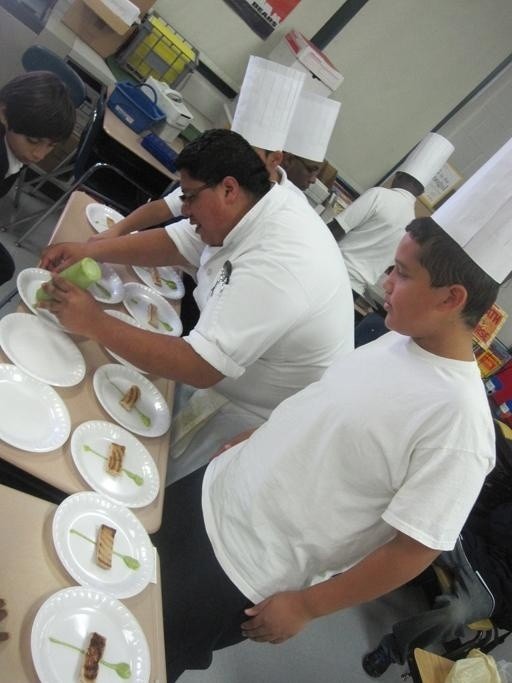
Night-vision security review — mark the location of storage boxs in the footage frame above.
[61,0,158,58]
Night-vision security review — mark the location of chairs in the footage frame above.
[401,648,455,683]
[0,43,87,232]
[12,95,179,248]
[399,560,497,677]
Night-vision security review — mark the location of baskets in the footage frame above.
[140,130,184,173]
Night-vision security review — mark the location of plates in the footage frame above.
[132,263,185,301]
[28,586,152,683]
[1,311,87,387]
[70,419,161,507]
[86,261,125,305]
[92,364,172,439]
[85,203,140,233]
[1,363,72,454]
[102,309,153,375]
[17,267,71,334]
[51,490,156,601]
[121,282,183,338]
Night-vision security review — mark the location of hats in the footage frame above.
[231,53,307,152]
[283,88,343,163]
[429,132,512,285]
[397,130,455,191]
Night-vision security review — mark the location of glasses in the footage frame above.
[178,183,219,203]
[294,155,322,173]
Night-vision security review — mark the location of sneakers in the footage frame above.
[361,648,391,679]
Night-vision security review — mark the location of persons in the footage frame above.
[150,135,512,683]
[0,70,77,286]
[363,497,511,679]
[324,131,455,304]
[281,91,342,193]
[33,127,356,491]
[86,54,314,289]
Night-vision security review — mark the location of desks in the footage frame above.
[64,86,182,221]
